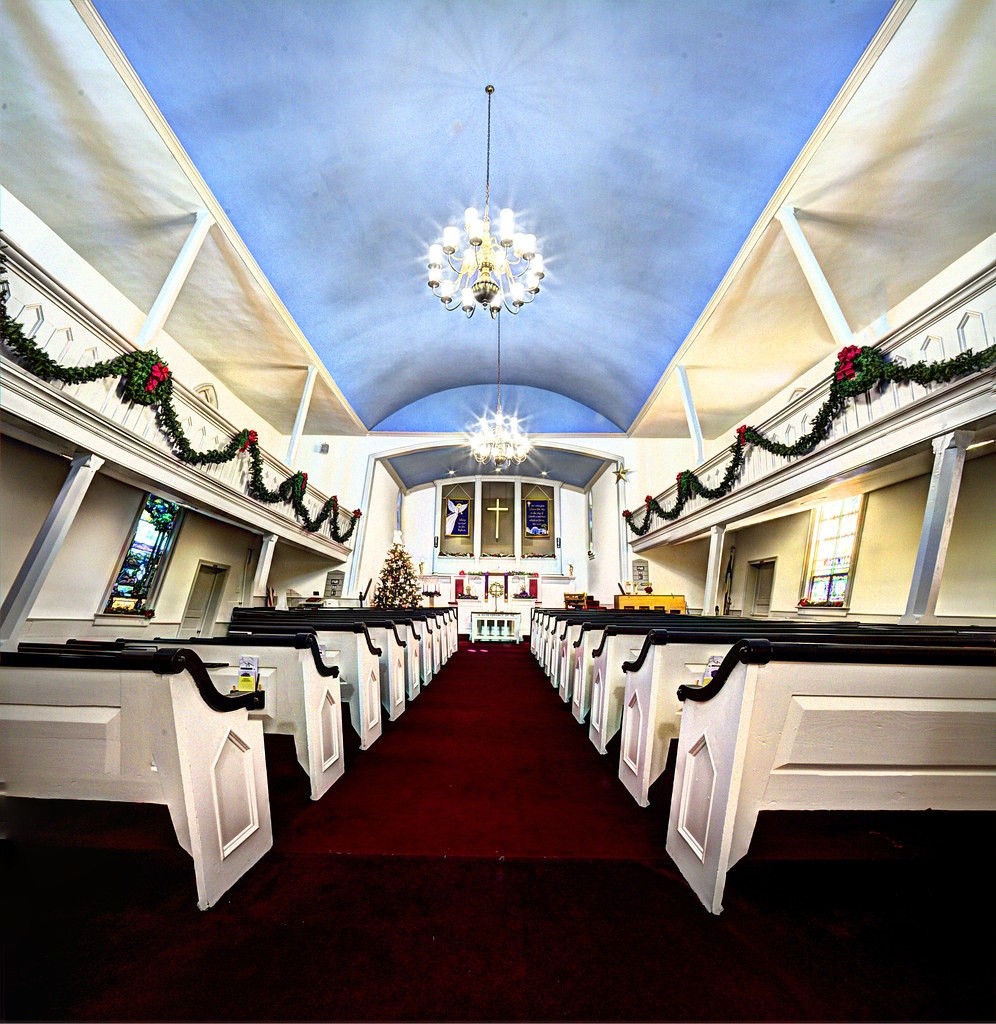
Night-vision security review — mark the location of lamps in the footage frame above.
[469,311,533,468]
[427,84,547,319]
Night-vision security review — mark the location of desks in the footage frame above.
[471,611,521,644]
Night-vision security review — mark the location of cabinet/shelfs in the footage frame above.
[615,595,686,614]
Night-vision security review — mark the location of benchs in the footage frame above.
[529,604,994,916]
[0,606,459,911]
